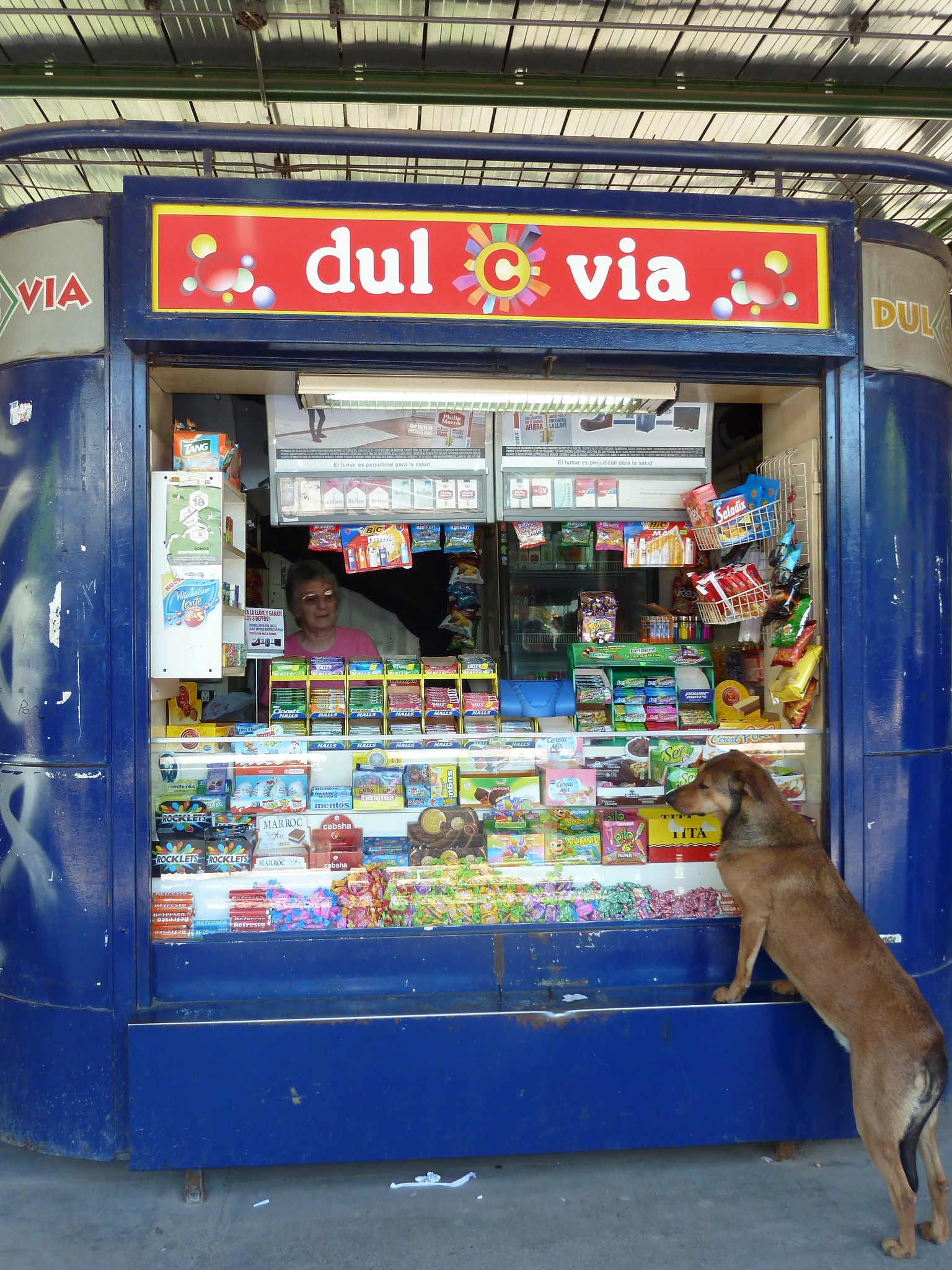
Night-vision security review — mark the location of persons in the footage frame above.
[252,559,383,723]
[307,409,327,443]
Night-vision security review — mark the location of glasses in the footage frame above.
[292,588,337,606]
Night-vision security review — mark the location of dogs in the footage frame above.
[661,749,951,1261]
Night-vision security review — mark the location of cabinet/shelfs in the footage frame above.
[149,470,250,682]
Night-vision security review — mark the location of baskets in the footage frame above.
[686,494,780,550]
[693,577,775,626]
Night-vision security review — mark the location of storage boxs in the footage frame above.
[148,724,812,868]
[706,493,754,545]
[680,482,723,549]
